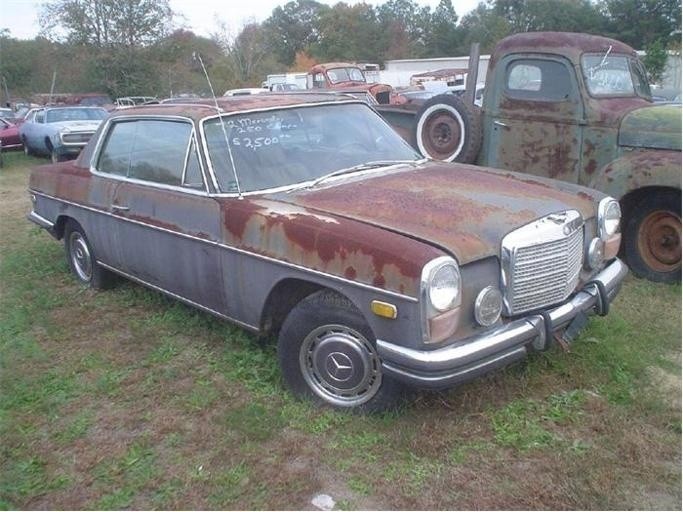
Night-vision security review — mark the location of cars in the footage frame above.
[27,92,631,417]
[0,96,173,164]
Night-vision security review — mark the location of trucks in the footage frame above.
[369,31,682,286]
[303,62,393,104]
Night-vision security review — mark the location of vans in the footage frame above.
[221,88,271,97]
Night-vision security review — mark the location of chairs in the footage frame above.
[210,120,302,196]
